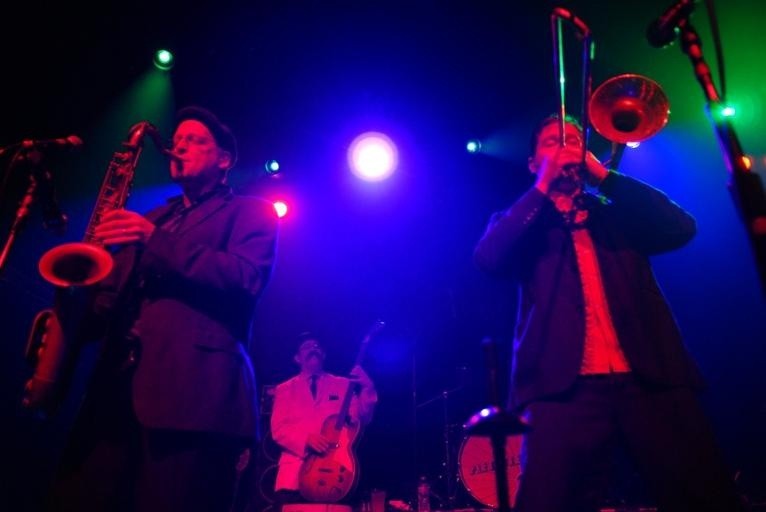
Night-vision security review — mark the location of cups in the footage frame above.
[371,489,385,510]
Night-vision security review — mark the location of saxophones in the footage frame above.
[29,117,172,410]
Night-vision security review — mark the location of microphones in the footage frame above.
[644,0,695,48]
[12,134,84,159]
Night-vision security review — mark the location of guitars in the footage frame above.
[298,321,387,502]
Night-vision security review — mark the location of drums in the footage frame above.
[458,407,528,507]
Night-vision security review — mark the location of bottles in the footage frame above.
[418,475,430,510]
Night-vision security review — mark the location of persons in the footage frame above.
[29,104,282,511]
[469,113,742,511]
[268,333,378,512]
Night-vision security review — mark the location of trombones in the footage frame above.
[552,8,670,193]
